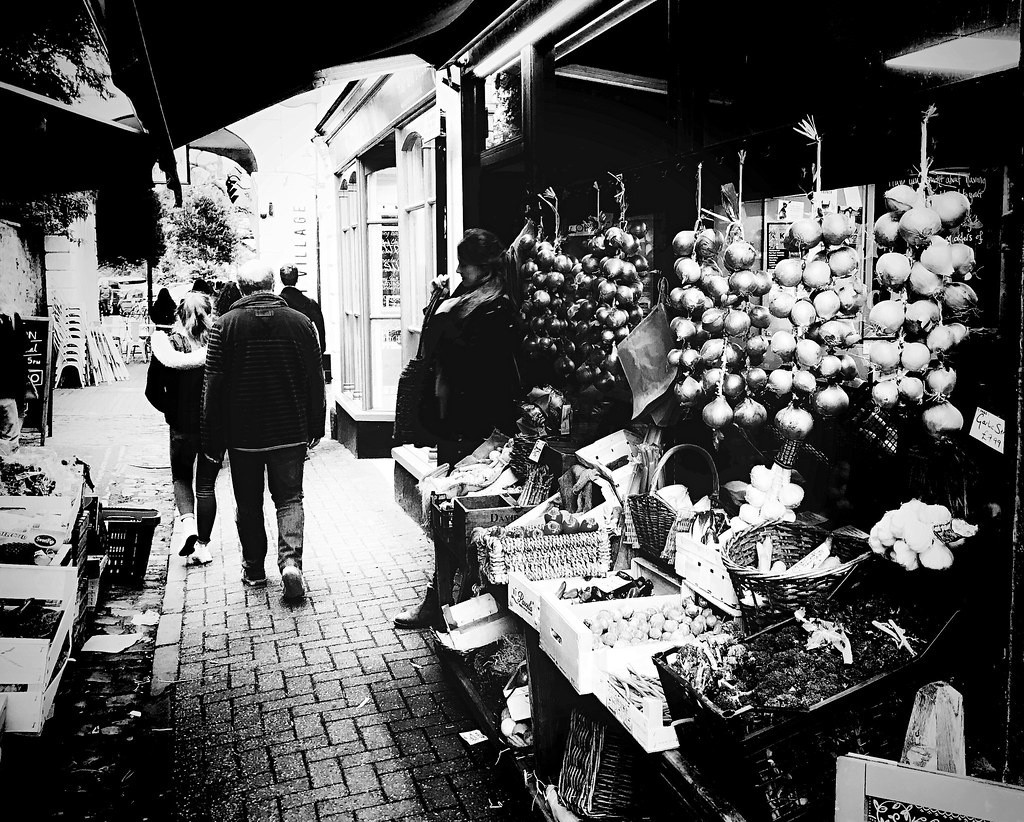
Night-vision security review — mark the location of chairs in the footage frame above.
[47,288,150,390]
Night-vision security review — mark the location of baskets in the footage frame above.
[557,708,652,822]
[99,507,162,585]
[628,445,727,564]
[722,521,875,636]
[476,521,612,584]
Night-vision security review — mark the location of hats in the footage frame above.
[193,278,211,294]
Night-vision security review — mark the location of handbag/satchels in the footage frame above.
[392,355,435,443]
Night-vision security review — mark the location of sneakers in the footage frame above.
[188,545,212,565]
[177,513,197,556]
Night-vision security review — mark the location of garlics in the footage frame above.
[729,463,804,532]
[868,500,954,571]
[670,188,977,439]
[520,232,643,388]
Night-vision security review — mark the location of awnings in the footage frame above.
[0,82,159,202]
[83,0,472,208]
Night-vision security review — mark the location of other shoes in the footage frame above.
[241,563,268,587]
[281,566,306,599]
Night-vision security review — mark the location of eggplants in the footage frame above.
[490,508,599,537]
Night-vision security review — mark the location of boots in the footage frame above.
[394,584,456,628]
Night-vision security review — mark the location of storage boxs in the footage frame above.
[0,507,161,735]
[508,572,974,822]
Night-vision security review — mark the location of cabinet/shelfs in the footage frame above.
[429,504,747,822]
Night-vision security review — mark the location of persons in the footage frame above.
[393,229,520,630]
[145,260,326,600]
